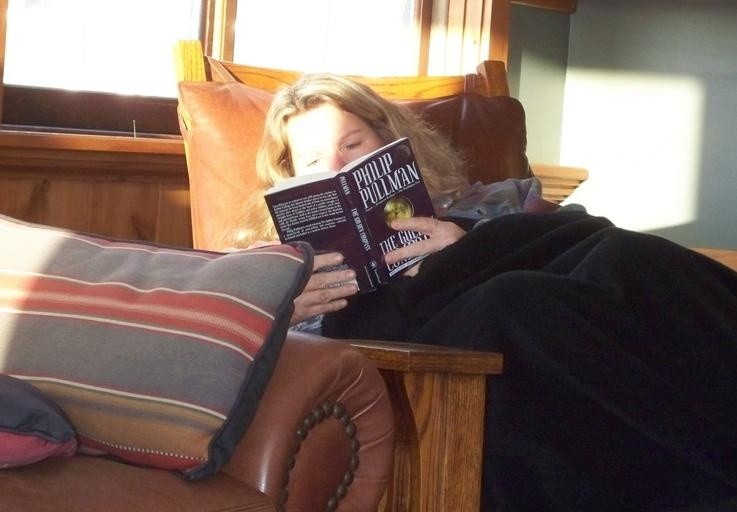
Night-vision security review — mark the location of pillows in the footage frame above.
[0,370,79,471]
[1,213,313,482]
[175,79,526,250]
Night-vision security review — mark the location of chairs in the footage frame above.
[173,36,737,511]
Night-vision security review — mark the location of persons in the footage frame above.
[219,70,587,334]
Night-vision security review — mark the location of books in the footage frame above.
[263,136,439,300]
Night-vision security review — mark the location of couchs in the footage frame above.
[0,330,397,512]
